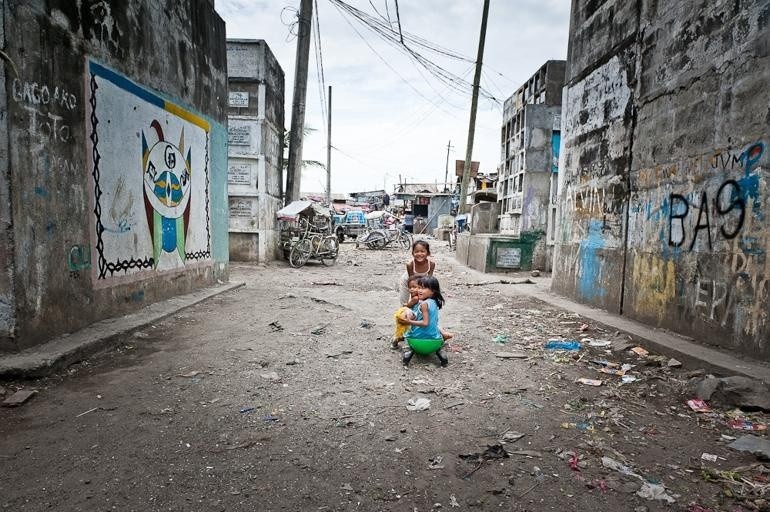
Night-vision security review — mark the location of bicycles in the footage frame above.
[357,221,415,250]
[289,217,339,269]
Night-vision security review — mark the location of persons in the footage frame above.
[398,239,436,306]
[396,276,446,340]
[390,272,453,350]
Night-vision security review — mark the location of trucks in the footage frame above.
[336,211,366,243]
